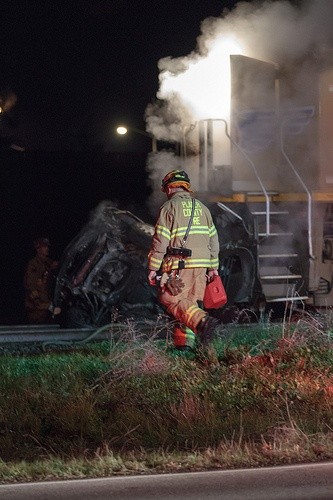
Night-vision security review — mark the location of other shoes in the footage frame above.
[175,345,194,354]
[198,315,221,346]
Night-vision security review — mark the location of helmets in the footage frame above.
[32,238,50,249]
[159,170,190,192]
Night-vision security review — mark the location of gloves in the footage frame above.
[159,273,184,296]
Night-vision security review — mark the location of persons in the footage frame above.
[24,237,52,323]
[149,169,220,350]
